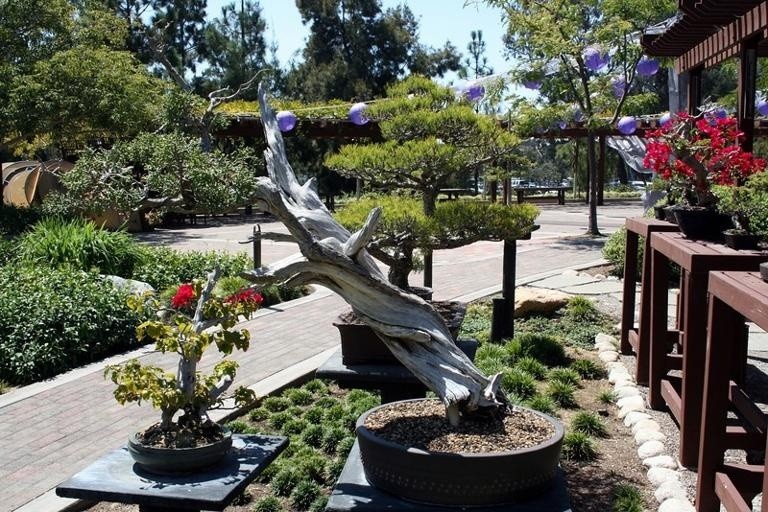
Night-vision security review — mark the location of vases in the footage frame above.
[355,393,565,510]
[128,419,233,471]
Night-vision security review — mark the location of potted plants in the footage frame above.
[641,102,768,284]
[320,71,542,366]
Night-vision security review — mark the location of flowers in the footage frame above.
[104,268,263,435]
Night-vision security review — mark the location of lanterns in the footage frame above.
[758,96,768,116]
[348,101,372,125]
[462,45,727,134]
[276,109,296,132]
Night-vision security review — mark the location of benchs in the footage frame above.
[515,186,573,205]
[439,187,472,201]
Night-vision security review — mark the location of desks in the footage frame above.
[315,336,480,406]
[650,230,767,464]
[326,437,566,511]
[55,432,292,511]
[692,268,767,511]
[622,216,678,388]
